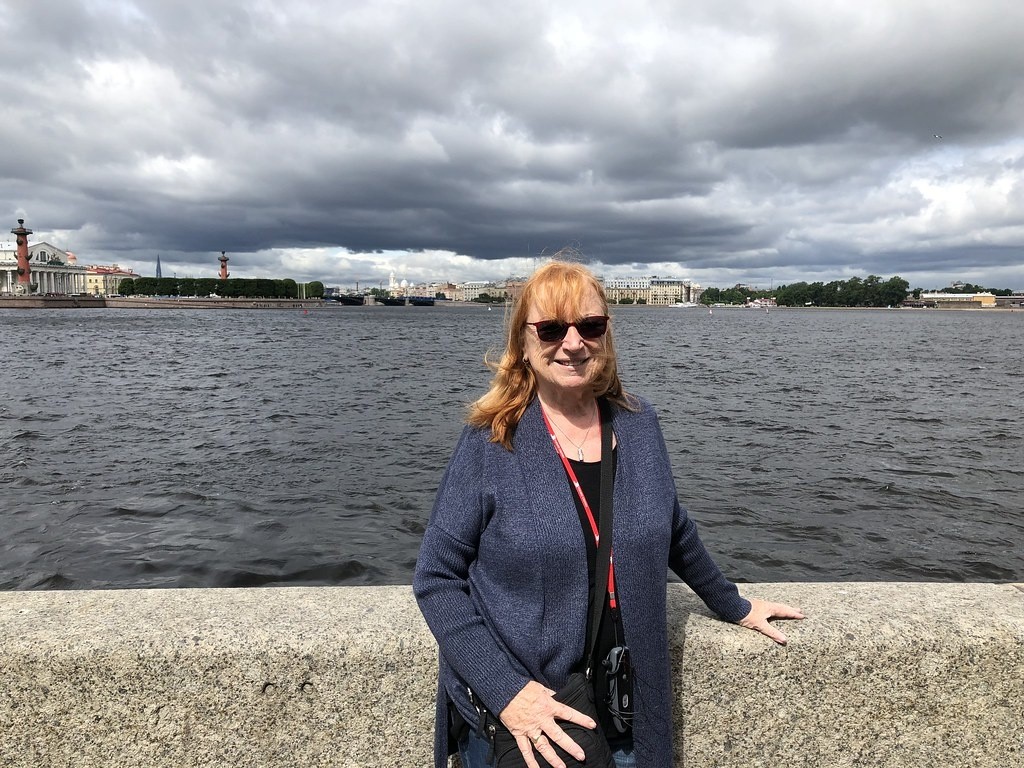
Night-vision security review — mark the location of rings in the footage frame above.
[531,733,541,745]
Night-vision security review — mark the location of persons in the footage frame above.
[413,260,804,768]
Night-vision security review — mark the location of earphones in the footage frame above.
[602,660,612,671]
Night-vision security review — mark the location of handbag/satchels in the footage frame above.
[474,671,617,768]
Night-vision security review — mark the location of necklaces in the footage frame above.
[543,403,597,461]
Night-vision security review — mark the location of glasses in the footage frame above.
[519,314,611,343]
